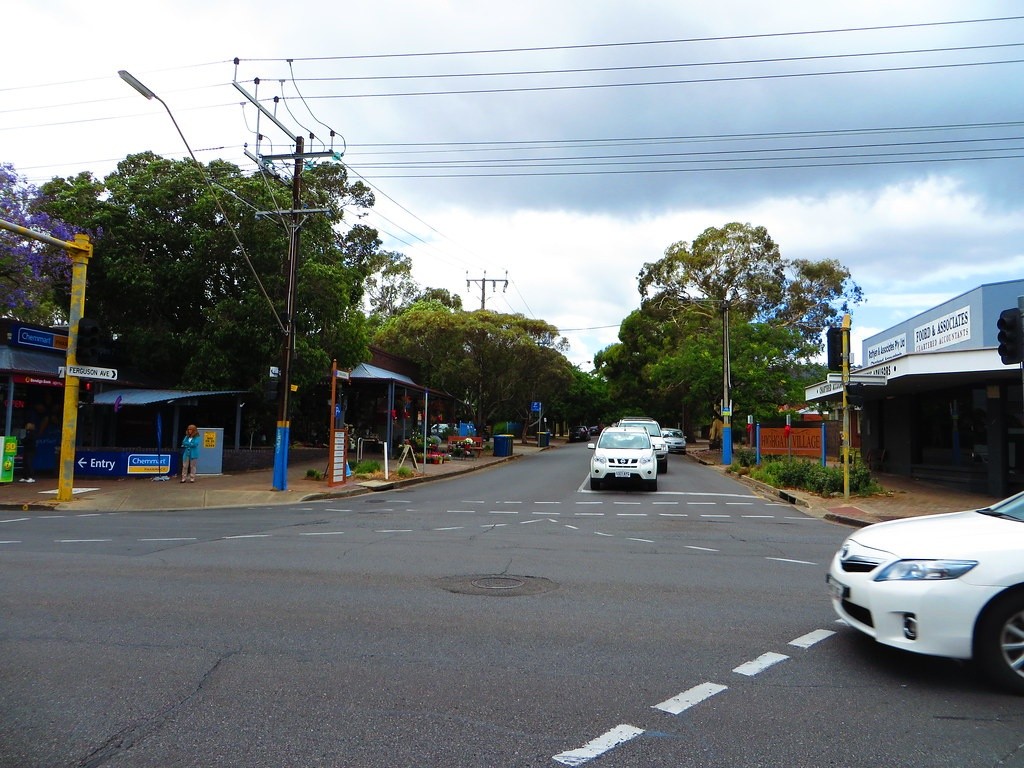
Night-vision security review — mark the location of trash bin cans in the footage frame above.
[493,434,514,457]
[537,432,550,447]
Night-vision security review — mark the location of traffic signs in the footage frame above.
[67,366,118,381]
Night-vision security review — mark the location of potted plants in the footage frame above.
[453,448,464,460]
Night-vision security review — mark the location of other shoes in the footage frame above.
[180,480,186,483]
[26,478,36,483]
[190,479,195,483]
[19,478,25,482]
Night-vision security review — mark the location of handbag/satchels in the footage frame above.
[176,447,186,457]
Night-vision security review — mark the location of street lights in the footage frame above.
[677,297,732,465]
[117,68,292,492]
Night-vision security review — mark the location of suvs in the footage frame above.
[569,426,590,442]
[587,426,658,491]
[617,417,668,474]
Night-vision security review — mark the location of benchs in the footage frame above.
[447,436,484,459]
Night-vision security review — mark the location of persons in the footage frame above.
[18,418,37,484]
[180,424,201,484]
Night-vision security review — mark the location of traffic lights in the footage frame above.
[998,307,1024,367]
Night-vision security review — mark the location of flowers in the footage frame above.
[416,452,452,461]
[463,437,473,445]
[431,435,442,444]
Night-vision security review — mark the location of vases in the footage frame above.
[431,444,438,448]
[434,460,439,464]
[443,461,450,464]
[439,460,443,464]
[466,444,471,449]
[418,458,424,463]
[426,459,430,464]
[431,459,434,464]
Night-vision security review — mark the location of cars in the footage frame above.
[660,428,687,454]
[825,490,1024,693]
[589,426,601,436]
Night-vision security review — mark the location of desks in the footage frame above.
[426,447,438,454]
